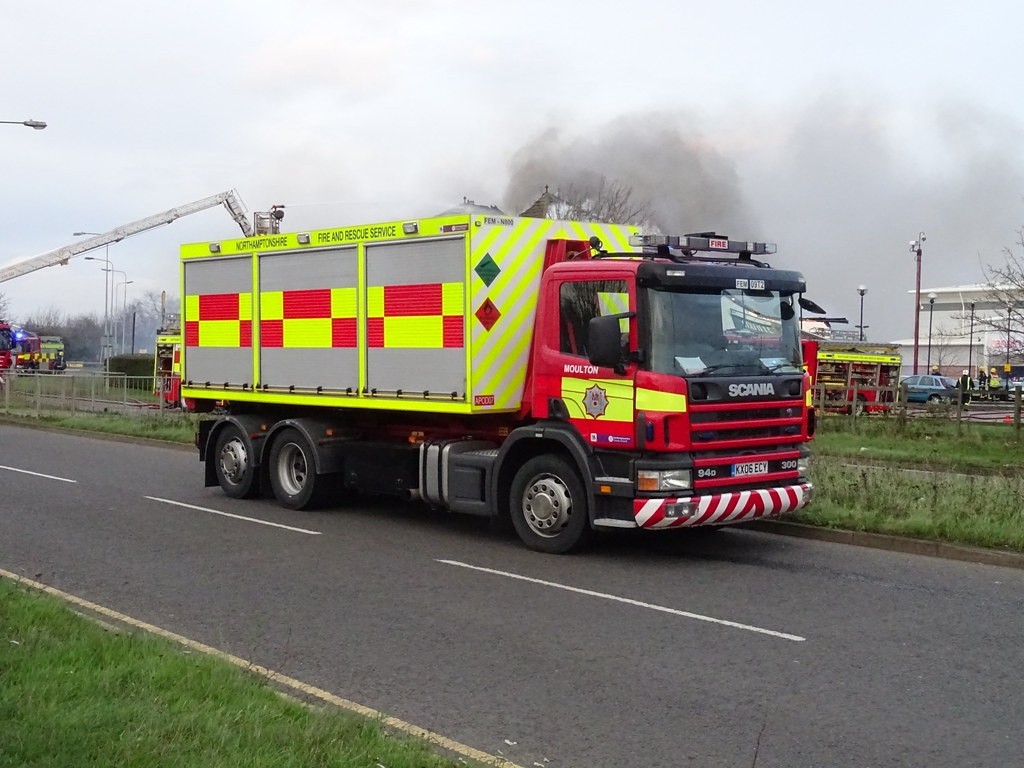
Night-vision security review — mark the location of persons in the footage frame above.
[978,366,986,399]
[955,370,975,411]
[52,351,67,370]
[987,368,999,400]
[931,366,941,376]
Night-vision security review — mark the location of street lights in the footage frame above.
[1005,303,1014,389]
[101,268,126,355]
[84,257,114,357]
[857,284,868,341]
[114,281,134,354]
[966,298,977,377]
[72,232,110,394]
[926,292,937,374]
[908,240,923,375]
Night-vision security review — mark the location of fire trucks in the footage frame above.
[0,189,285,376]
[739,337,900,417]
[179,214,823,553]
[153,312,195,412]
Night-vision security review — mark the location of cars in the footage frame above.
[898,375,964,404]
[969,380,1024,401]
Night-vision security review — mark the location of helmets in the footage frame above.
[963,370,969,375]
[932,365,938,369]
[979,366,984,371]
[990,368,996,373]
